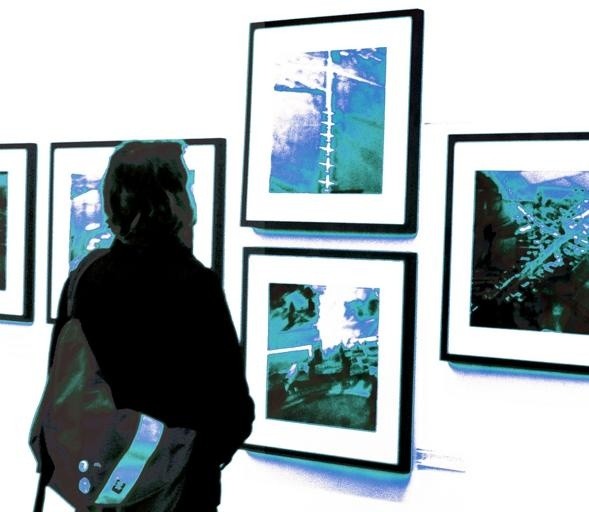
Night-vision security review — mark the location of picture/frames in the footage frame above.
[237,244,419,478]
[0,139,38,326]
[44,137,229,326]
[440,133,588,373]
[239,7,428,238]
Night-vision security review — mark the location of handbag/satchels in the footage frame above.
[28,318,197,512]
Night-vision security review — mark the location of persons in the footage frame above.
[48,142,255,512]
